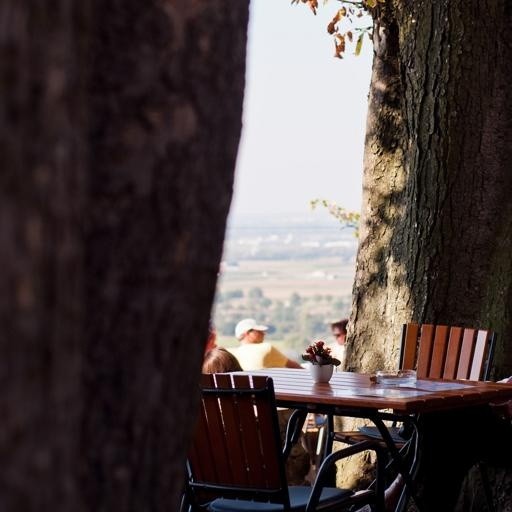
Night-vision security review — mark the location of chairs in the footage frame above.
[184,374,385,512]
[330,324,497,512]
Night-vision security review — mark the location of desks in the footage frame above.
[213,368,512,512]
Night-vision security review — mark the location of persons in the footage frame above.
[227,317,304,370]
[382,377,511,511]
[330,317,348,372]
[201,345,312,486]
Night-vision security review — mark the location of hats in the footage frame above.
[235,319,268,339]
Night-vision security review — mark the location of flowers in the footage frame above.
[302,341,341,366]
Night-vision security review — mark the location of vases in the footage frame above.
[310,364,333,384]
